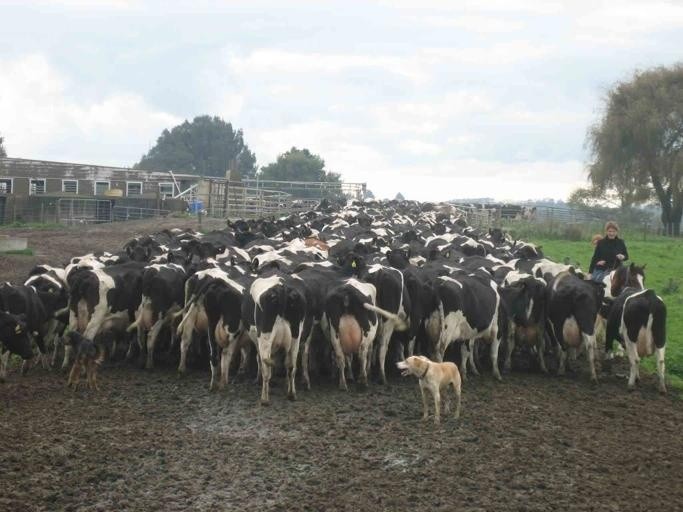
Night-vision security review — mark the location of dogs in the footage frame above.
[395,355,462,425]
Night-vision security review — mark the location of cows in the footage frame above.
[603,286,667,395]
[0,198,607,407]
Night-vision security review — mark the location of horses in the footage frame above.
[574,262,647,354]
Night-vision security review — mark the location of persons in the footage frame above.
[589,221,629,284]
